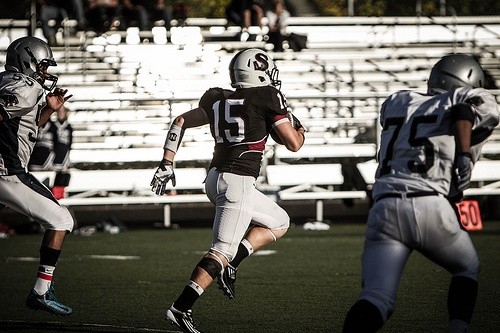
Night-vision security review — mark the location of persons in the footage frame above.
[149,47,306,333]
[37,0,62,46]
[0,36,74,315]
[0,104,73,240]
[226,0,290,35]
[88,0,188,34]
[341,55,500,333]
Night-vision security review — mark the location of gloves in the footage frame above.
[150,158,176,195]
[452,152,473,191]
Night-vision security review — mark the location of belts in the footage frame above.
[375,190,439,203]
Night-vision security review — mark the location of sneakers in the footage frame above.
[217,263,238,299]
[165,302,201,333]
[25,280,73,315]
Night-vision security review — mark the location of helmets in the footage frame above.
[5,36,53,85]
[426,55,485,96]
[229,49,276,88]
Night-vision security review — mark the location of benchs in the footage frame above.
[0,18,500,230]
